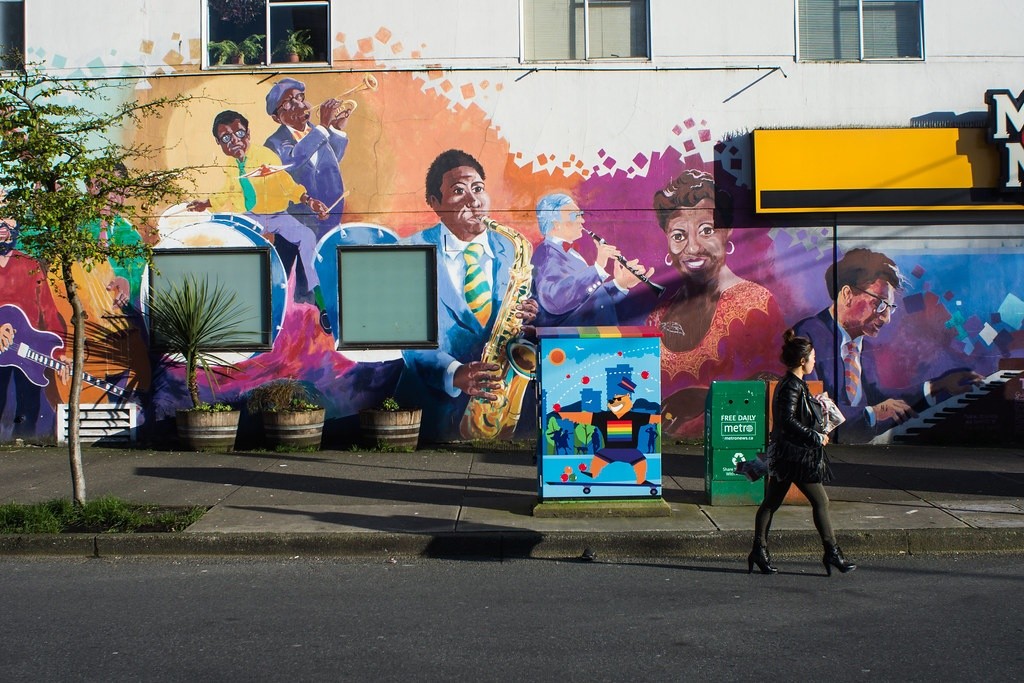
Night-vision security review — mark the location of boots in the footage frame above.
[747,542,778,575]
[821,536,856,576]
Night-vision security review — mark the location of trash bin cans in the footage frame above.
[704,380,768,506]
[769,379,825,506]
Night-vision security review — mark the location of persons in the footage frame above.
[747,329,856,576]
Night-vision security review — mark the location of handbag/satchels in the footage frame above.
[814,394,846,435]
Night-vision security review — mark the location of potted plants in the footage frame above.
[135,271,273,451]
[272,29,314,63]
[258,375,326,451]
[360,396,424,451]
[208,34,266,66]
